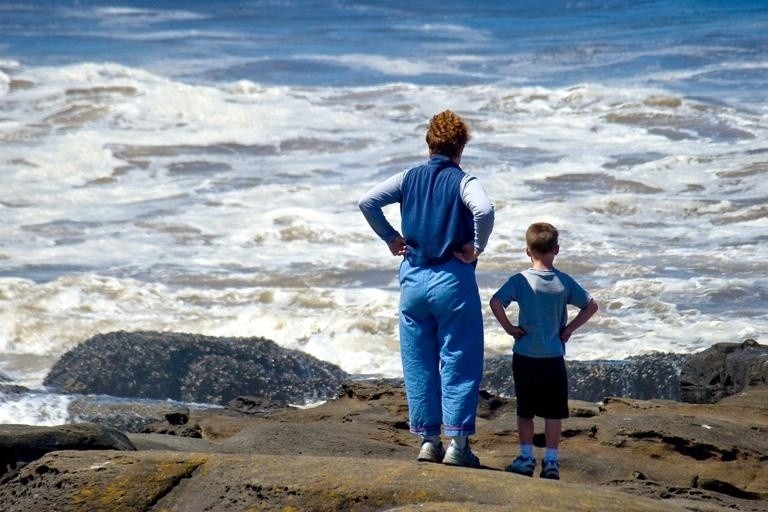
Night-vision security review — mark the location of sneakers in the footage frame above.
[505,455,536,477]
[540,458,561,479]
[417,438,445,463]
[441,444,480,467]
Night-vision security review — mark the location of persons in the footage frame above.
[354,108,495,467]
[488,221,599,481]
